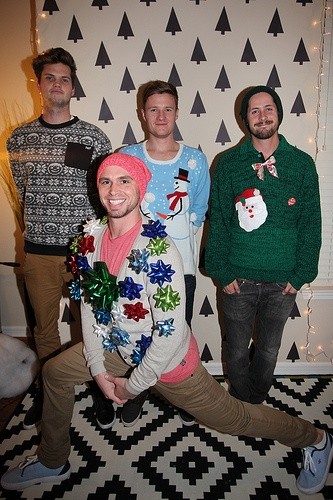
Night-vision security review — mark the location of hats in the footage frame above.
[96,152,151,203]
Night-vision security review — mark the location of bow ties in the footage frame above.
[251,156,279,182]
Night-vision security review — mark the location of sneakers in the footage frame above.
[119,389,143,427]
[22,389,45,430]
[89,392,115,430]
[296,431,332,494]
[1,454,72,490]
[176,404,197,426]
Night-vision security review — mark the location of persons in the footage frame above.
[1,151,333,500]
[114,79,213,427]
[6,48,116,430]
[198,85,323,407]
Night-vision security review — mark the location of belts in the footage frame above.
[236,278,275,287]
